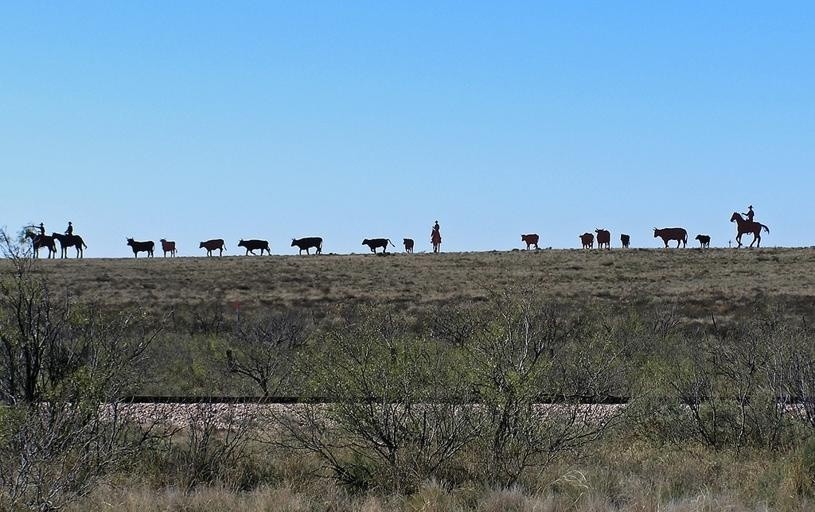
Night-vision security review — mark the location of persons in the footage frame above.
[35,222,45,236]
[64,221,73,236]
[432,219,440,232]
[741,205,755,222]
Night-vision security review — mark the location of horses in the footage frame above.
[51,232,87,259]
[730,212,769,248]
[24,229,57,259]
[430,229,441,253]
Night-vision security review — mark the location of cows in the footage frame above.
[160,237,177,257]
[653,226,688,248]
[126,236,154,258]
[291,237,323,255]
[238,238,272,256]
[595,228,610,249]
[579,232,594,249]
[620,234,630,248]
[362,238,395,254]
[521,233,539,250]
[199,239,227,257]
[695,234,710,248]
[402,238,414,254]
